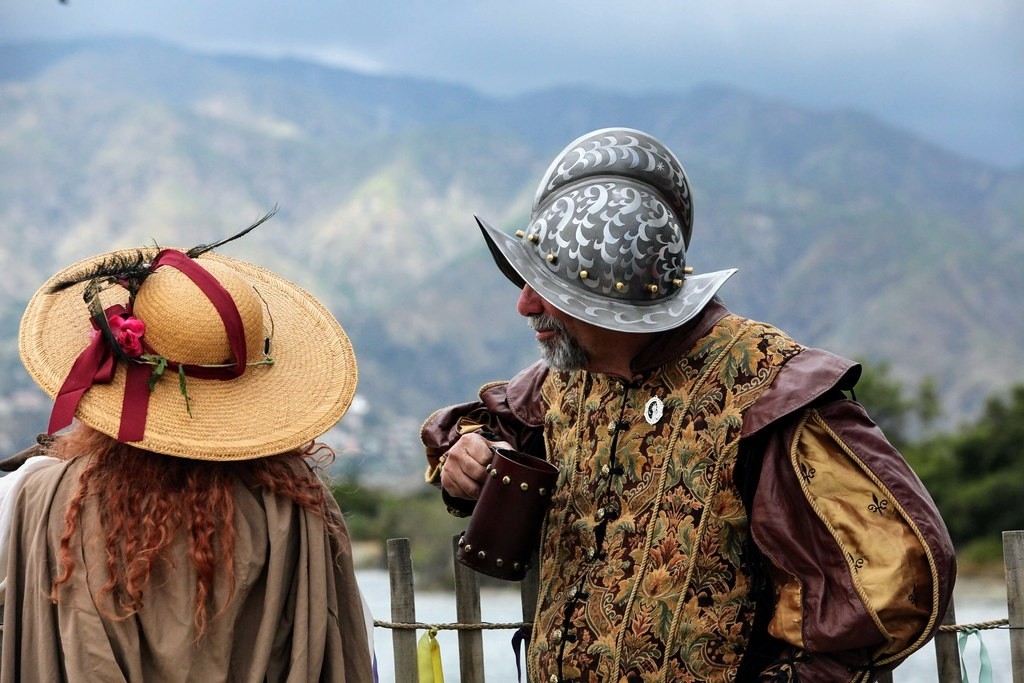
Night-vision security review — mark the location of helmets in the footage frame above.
[472,128,739,333]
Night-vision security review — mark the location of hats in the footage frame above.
[19,206,356,461]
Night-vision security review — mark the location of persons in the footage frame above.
[0,248,375,683]
[419,127,957,683]
